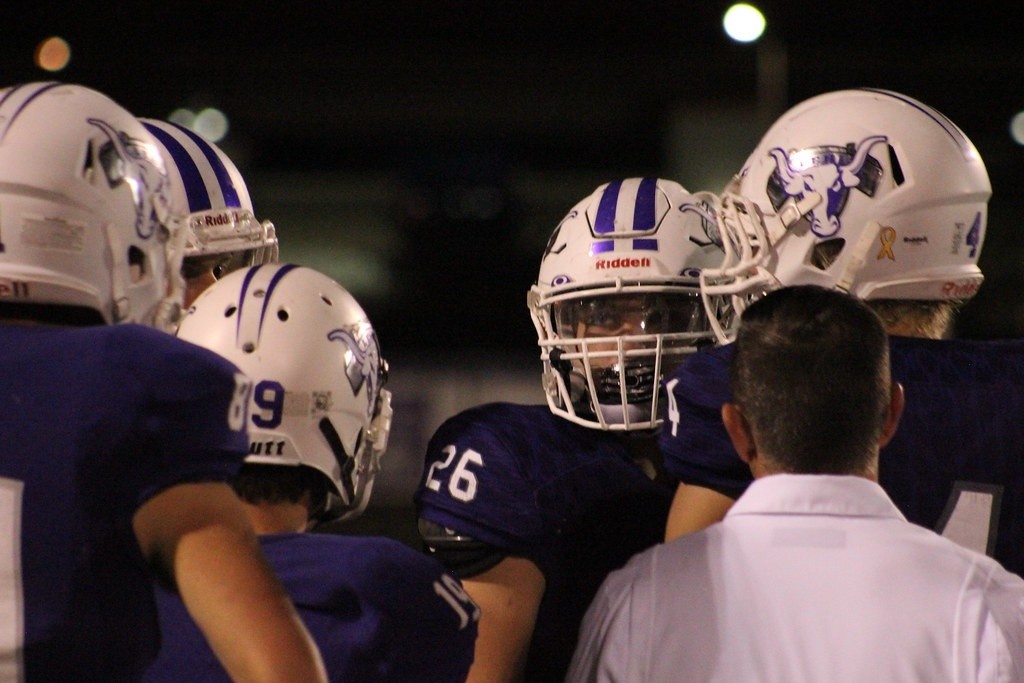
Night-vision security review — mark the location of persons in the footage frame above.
[661,87,1024,580]
[0,82,329,683]
[565,284,1024,683]
[152,262,481,683]
[414,176,741,683]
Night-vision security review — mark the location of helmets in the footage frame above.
[134,117,278,265]
[0,82,190,334]
[176,264,391,522]
[693,87,993,345]
[527,177,742,431]
